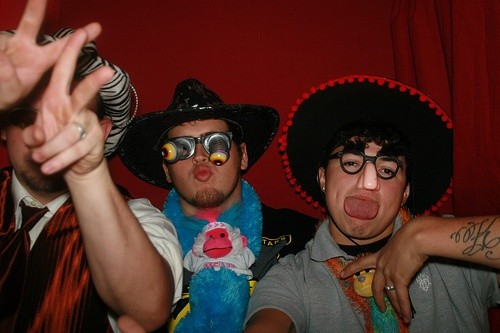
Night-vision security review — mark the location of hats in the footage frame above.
[277,76,454,216]
[120,79,280,189]
[0,27,137,158]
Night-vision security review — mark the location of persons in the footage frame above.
[117,77,321,332]
[0,0,184,333]
[243,75,499,333]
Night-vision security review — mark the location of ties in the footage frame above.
[0,201,48,333]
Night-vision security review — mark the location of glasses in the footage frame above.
[10,107,37,128]
[326,148,405,179]
[159,132,232,166]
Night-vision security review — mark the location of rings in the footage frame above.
[67,120,85,139]
[386,285,394,290]
